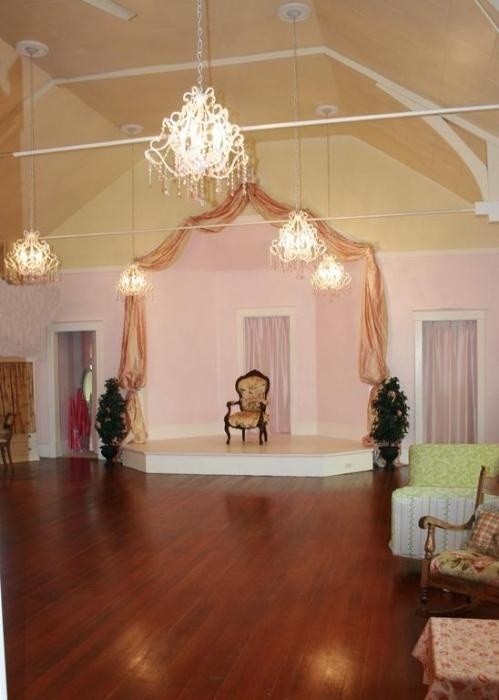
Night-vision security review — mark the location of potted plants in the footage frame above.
[95,377,131,461]
[367,376,411,470]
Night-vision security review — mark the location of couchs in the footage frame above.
[388,442,499,560]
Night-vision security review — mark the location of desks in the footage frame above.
[415,615,498,699]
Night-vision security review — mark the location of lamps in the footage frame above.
[0,37,63,288]
[114,121,151,300]
[271,2,348,294]
[147,0,251,208]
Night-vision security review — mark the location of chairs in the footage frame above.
[0,411,18,471]
[221,369,271,446]
[417,465,499,619]
